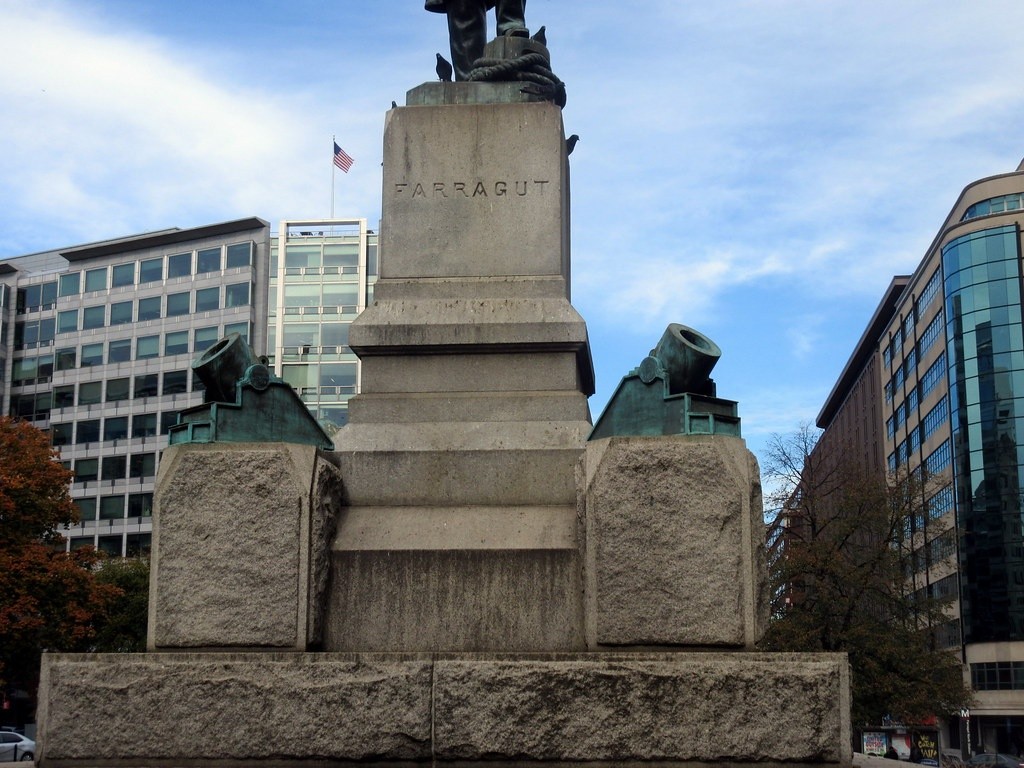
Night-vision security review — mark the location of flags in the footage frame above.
[334,142,354,173]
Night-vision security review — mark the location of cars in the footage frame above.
[965,753,1024,768]
[0,731,35,761]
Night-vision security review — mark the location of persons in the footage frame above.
[908,743,923,764]
[866,732,884,752]
[884,746,898,760]
[424,0,529,82]
